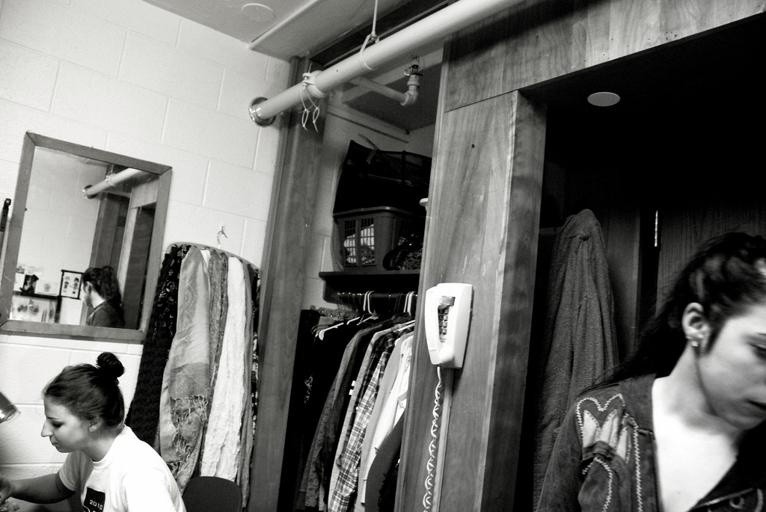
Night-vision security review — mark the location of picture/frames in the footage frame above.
[58,269,84,300]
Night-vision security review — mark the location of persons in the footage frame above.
[535,232,766,512]
[0,352,188,512]
[80,265,125,328]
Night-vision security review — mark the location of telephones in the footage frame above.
[424,282,474,370]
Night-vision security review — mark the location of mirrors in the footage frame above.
[0,131,172,345]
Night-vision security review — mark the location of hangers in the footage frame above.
[300,291,418,354]
[165,229,261,277]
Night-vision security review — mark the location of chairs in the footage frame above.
[182,476,242,511]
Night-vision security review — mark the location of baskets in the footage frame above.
[333,206,411,271]
